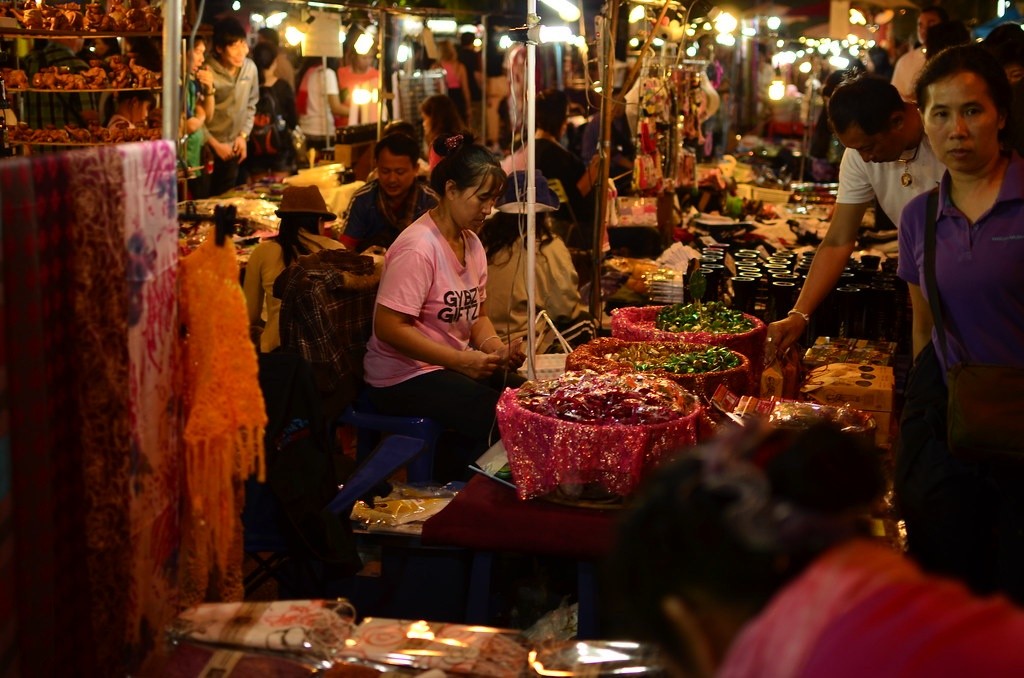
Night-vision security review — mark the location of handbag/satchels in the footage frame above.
[948,363,1024,455]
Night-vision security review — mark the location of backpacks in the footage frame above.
[241,91,289,172]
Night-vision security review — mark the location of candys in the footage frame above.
[520,299,756,430]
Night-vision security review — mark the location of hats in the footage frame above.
[492,169,560,213]
[275,185,336,222]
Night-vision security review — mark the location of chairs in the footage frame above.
[282,249,443,520]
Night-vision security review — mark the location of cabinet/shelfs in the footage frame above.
[0,27,214,154]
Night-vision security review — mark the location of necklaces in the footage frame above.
[897,137,923,187]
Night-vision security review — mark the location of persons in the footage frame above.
[614,417,1024,678]
[93,19,507,200]
[246,92,633,493]
[766,0,1024,601]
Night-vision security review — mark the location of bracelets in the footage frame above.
[479,336,500,351]
[787,309,809,324]
